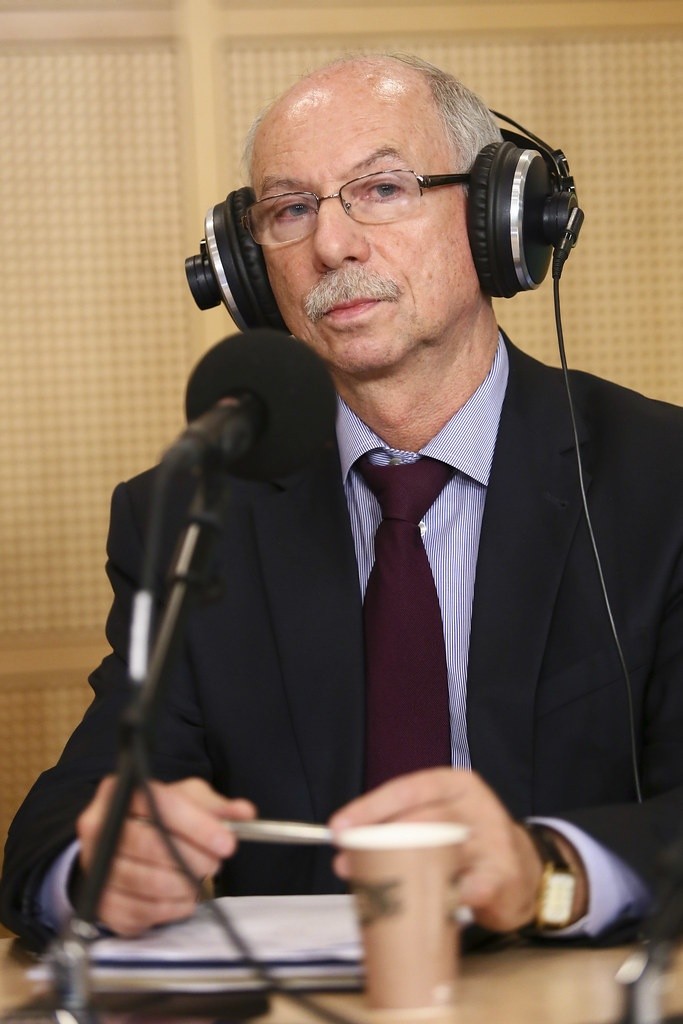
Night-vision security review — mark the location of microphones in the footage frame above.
[158,333,336,484]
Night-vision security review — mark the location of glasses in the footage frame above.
[241,168,471,245]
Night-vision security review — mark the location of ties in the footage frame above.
[355,457,459,794]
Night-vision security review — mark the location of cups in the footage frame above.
[332,824,470,1009]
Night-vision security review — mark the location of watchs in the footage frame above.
[495,828,574,942]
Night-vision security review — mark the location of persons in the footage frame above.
[3,46,680,954]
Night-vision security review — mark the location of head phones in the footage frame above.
[184,108,584,333]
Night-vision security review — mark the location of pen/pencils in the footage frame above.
[218,819,335,847]
